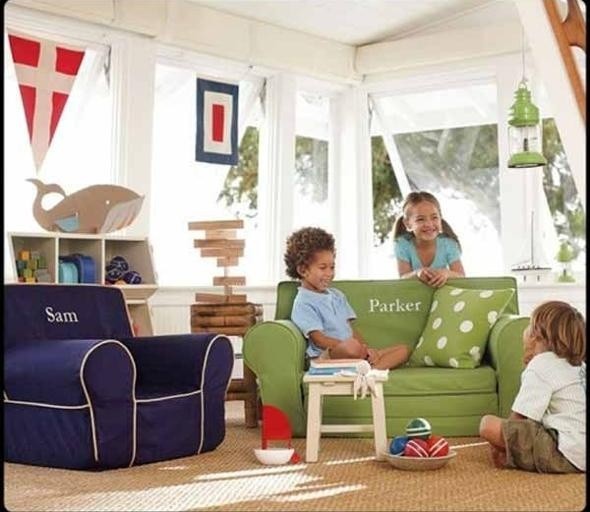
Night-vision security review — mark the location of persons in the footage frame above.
[393,192,465,288]
[479,301,588,474]
[285,227,411,372]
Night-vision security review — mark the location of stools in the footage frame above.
[302,370,388,463]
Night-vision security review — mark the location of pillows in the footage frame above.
[402,282,516,370]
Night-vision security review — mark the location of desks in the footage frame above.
[191,302,264,427]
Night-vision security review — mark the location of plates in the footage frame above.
[381,448,458,470]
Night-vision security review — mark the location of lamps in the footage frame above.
[507,26,547,168]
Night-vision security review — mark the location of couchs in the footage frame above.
[242,277,533,437]
[4,285,234,472]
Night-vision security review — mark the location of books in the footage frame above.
[307,358,370,376]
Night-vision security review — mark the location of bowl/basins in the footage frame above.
[254,446,294,466]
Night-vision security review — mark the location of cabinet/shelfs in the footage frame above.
[7,231,158,338]
